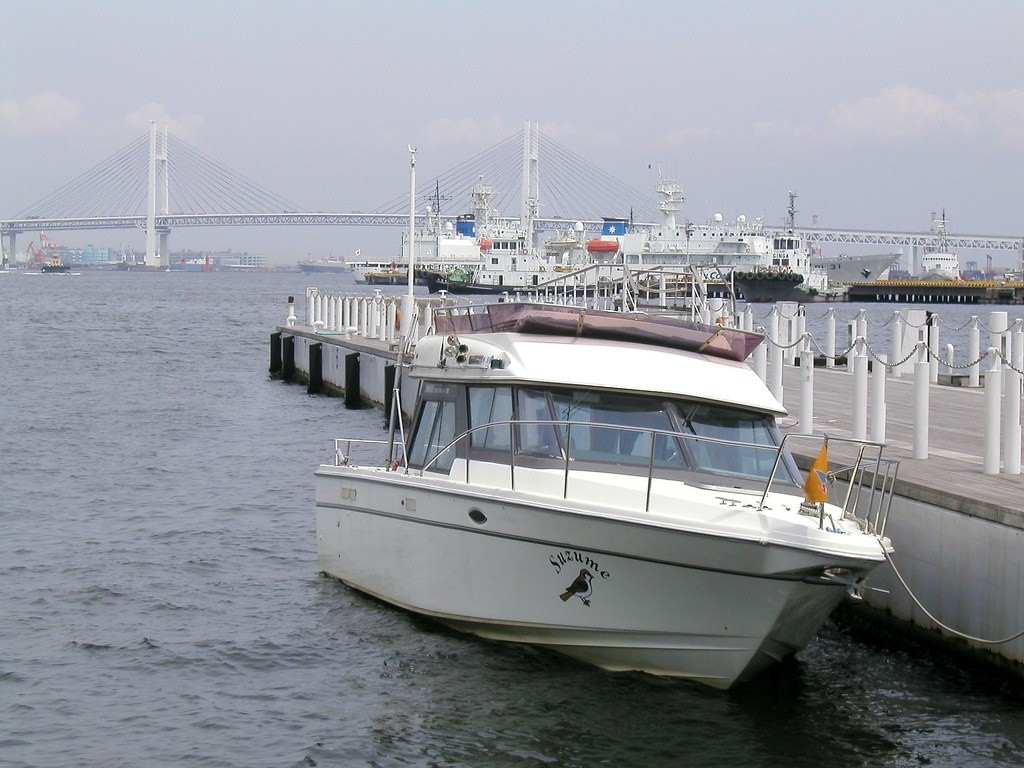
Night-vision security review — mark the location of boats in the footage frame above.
[40,255,71,274]
[312,301,901,692]
[298,169,1024,308]
[117,260,170,271]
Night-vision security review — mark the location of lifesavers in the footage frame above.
[395,308,401,331]
